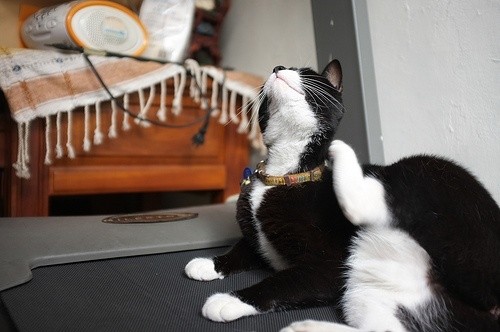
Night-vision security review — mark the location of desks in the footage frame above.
[0,49,265,216]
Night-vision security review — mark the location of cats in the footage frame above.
[184,59,500,332]
